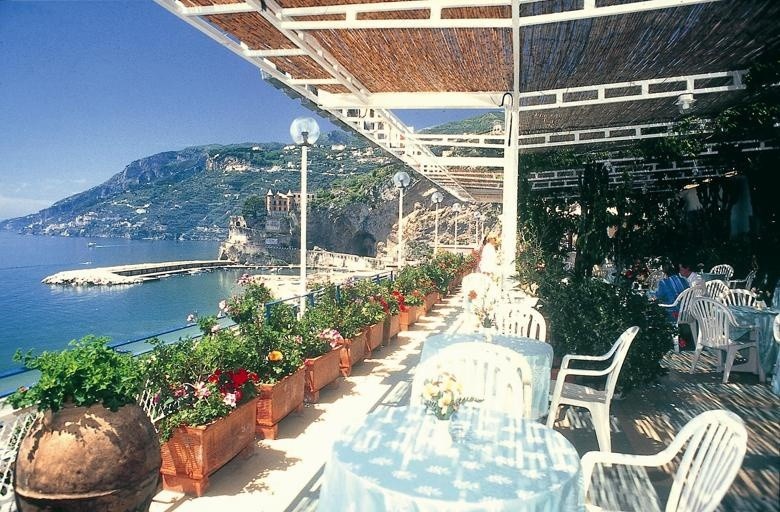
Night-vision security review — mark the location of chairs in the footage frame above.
[581,407,749,512]
[685,296,761,384]
[484,265,780,338]
[543,323,643,453]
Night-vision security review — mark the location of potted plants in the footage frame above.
[6,335,163,510]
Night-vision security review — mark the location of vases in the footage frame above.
[160,398,260,495]
[305,348,339,401]
[337,331,369,375]
[428,418,454,450]
[368,277,463,352]
[253,366,309,439]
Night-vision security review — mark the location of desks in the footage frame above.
[408,334,553,420]
[322,332,580,511]
[323,405,578,511]
[718,304,780,381]
[770,313,780,398]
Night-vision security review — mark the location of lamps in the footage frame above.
[676,95,693,111]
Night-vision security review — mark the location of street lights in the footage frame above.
[430,190,444,260]
[287,116,322,327]
[392,170,411,276]
[451,201,461,256]
[480,214,486,243]
[473,210,481,249]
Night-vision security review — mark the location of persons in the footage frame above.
[679,259,707,350]
[646,263,691,324]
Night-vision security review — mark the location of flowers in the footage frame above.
[293,329,344,348]
[416,364,464,419]
[159,367,258,440]
[267,349,283,363]
[339,255,463,324]
